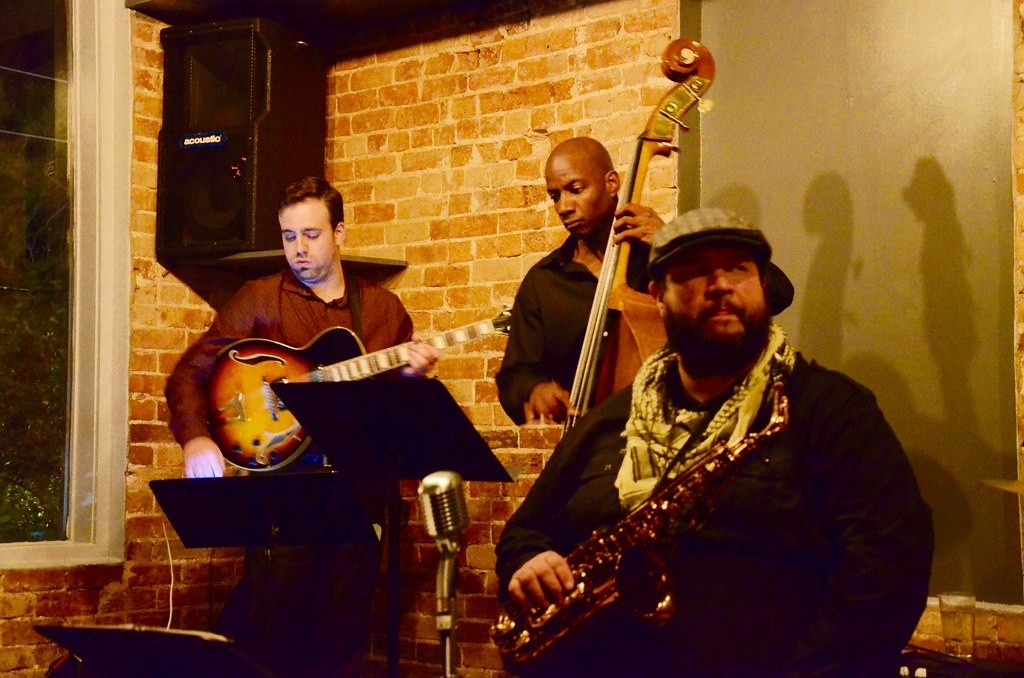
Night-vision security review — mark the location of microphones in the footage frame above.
[418,471,468,632]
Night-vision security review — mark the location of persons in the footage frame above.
[495,208,936,678]
[496,137,667,427]
[165,176,440,678]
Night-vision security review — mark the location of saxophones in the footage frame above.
[491,374,788,666]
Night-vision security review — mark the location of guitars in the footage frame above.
[207,311,514,471]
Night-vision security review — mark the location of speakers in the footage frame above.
[156,16,327,262]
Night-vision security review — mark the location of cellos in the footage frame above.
[563,39,713,439]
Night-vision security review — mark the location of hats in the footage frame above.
[649,209,794,316]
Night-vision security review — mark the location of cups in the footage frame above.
[939,593,975,657]
